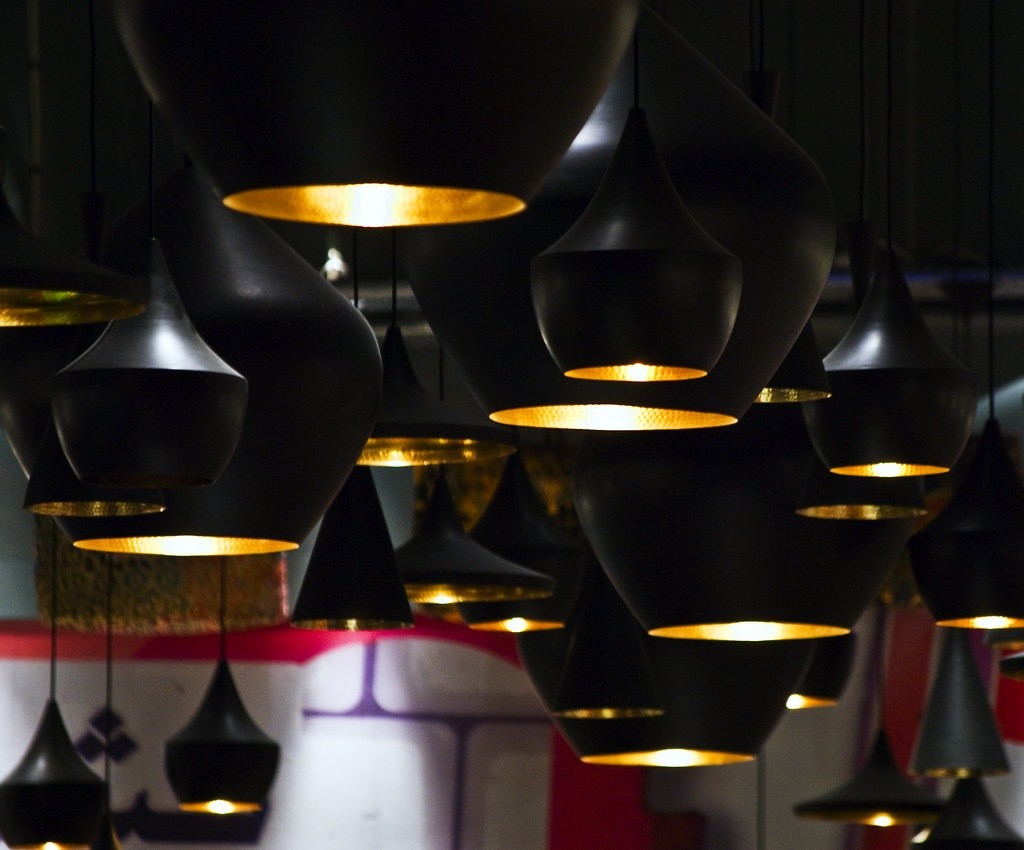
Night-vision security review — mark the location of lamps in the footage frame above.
[0,0,1024,768]
[792,602,943,827]
[907,623,1012,781]
[163,558,279,816]
[0,518,108,850]
[910,778,1024,850]
